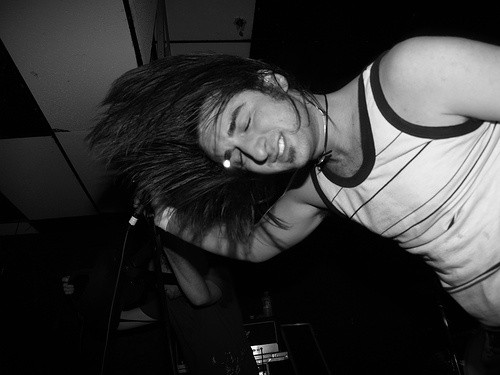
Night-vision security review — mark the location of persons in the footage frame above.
[79,33,500,375]
